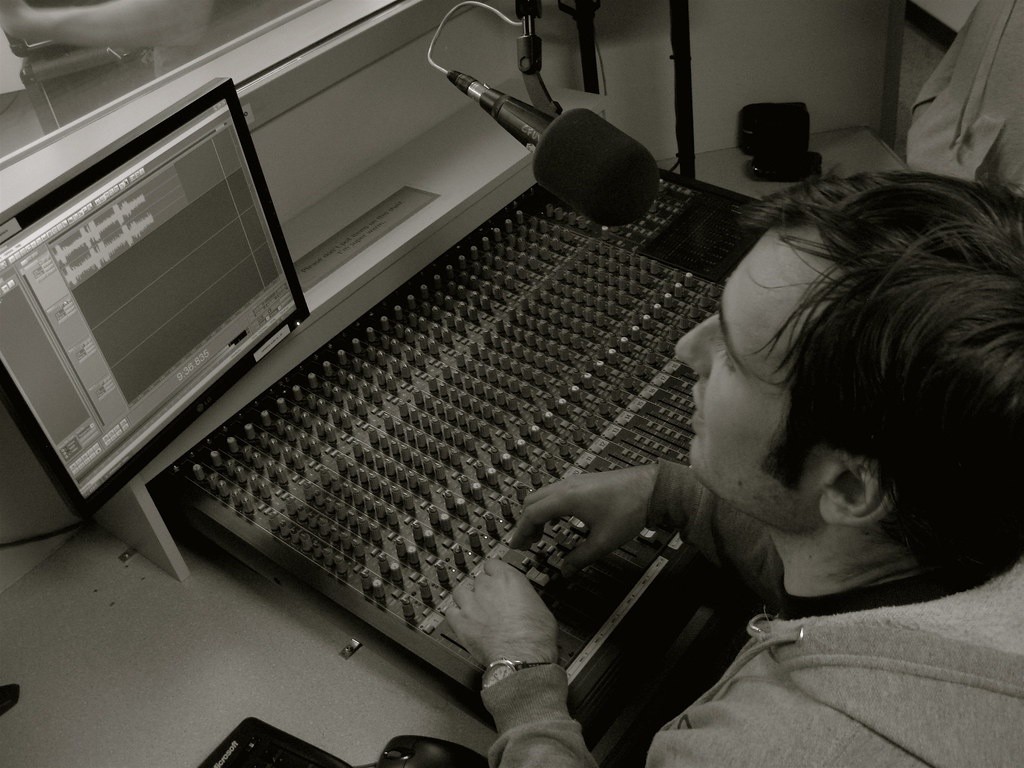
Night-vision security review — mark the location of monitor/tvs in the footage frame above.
[0,77,311,522]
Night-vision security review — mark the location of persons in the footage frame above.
[444,168,1024,768]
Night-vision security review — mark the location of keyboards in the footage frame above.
[197,716,353,768]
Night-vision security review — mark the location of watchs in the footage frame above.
[481,657,553,690]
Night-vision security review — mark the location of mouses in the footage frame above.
[378,734,491,768]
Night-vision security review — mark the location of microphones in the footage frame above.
[446,69,661,226]
